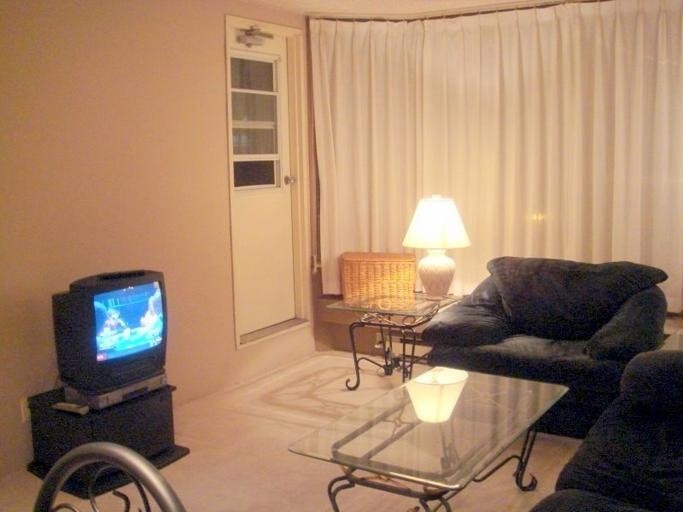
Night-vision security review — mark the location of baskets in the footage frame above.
[338,251,418,309]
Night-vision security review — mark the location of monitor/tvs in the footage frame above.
[52,271,168,396]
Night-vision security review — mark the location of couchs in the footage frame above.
[529,349,683,512]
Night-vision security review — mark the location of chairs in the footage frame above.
[421,257,668,438]
[32,440,184,512]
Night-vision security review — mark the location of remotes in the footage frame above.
[52,403,90,415]
[97,271,145,279]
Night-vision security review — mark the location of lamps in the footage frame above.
[402,194,471,296]
[405,366,469,423]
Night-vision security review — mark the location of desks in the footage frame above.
[27,385,190,499]
